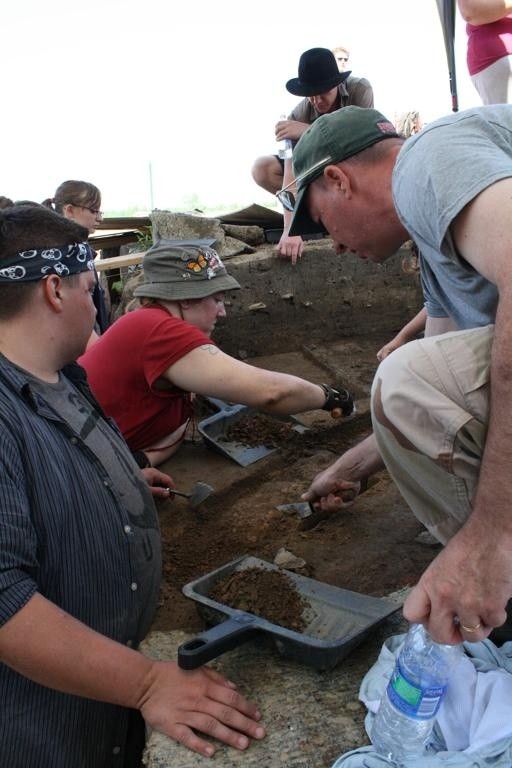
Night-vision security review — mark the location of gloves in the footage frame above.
[321,381,355,417]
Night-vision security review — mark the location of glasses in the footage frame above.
[71,203,105,217]
[275,155,333,211]
[337,56,348,62]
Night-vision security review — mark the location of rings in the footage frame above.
[458,622,481,634]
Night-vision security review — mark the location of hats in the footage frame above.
[287,105,398,237]
[285,47,352,98]
[133,243,241,301]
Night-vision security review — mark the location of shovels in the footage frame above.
[275,491,356,521]
[175,553,404,671]
[165,482,214,506]
[195,394,297,466]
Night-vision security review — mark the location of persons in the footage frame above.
[288,103,512,646]
[77,242,354,453]
[460,0,512,106]
[253,49,374,267]
[0,202,265,768]
[42,180,103,236]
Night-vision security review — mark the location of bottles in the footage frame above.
[369,622,461,764]
